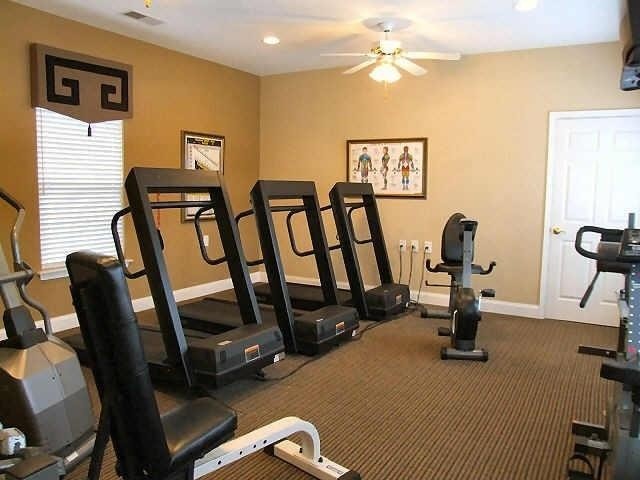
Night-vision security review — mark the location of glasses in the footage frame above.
[368,64,401,82]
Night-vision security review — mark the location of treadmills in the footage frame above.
[164,181,360,357]
[61,167,287,394]
[247,181,410,321]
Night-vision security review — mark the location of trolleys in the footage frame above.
[320,21,460,76]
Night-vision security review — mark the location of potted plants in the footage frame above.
[181,130,225,223]
[347,138,427,199]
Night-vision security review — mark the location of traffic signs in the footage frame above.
[399,240,433,254]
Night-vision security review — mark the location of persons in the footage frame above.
[356,147,373,183]
[381,147,390,191]
[397,146,414,190]
